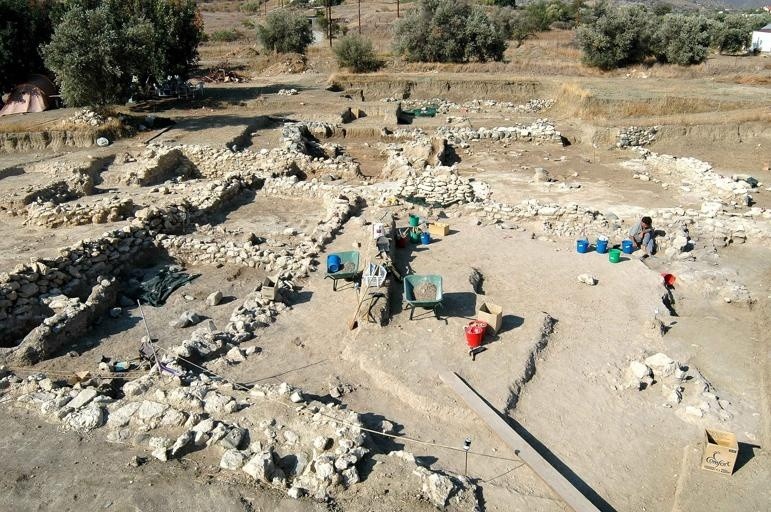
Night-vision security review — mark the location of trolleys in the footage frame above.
[323,250,359,292]
[403,274,445,320]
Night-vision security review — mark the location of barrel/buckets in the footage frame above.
[397,215,430,249]
[577,239,589,253]
[465,320,487,346]
[622,240,633,254]
[327,255,341,271]
[596,240,608,253]
[661,272,674,285]
[608,248,620,263]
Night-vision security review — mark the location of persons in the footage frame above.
[629,217,655,257]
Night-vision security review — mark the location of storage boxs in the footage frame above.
[477,301,503,334]
[700,427,739,477]
[428,221,449,236]
